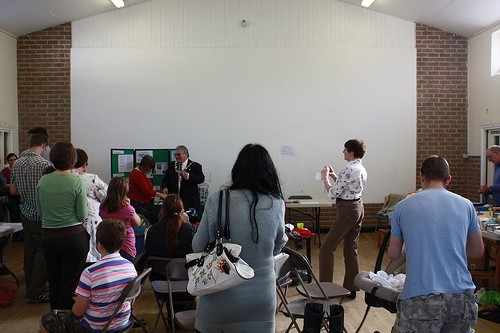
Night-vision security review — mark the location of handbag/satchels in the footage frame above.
[185,188,255,296]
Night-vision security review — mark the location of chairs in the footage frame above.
[354,229,398,333]
[275,246,351,333]
[371,193,407,240]
[102,252,195,333]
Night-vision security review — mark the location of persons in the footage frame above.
[479,145,500,207]
[163,146,205,224]
[0,126,108,312]
[99,176,141,264]
[318,140,367,299]
[192,144,286,333]
[128,154,168,223]
[145,194,199,333]
[387,155,484,333]
[40,219,137,333]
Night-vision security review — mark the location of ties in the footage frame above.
[179,162,182,176]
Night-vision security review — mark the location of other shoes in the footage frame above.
[31,293,49,301]
[347,291,356,299]
[40,286,50,295]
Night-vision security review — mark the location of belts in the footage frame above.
[337,197,360,202]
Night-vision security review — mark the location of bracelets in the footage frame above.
[323,180,329,183]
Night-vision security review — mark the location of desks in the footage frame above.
[0,223,24,288]
[286,199,333,248]
[286,228,316,283]
